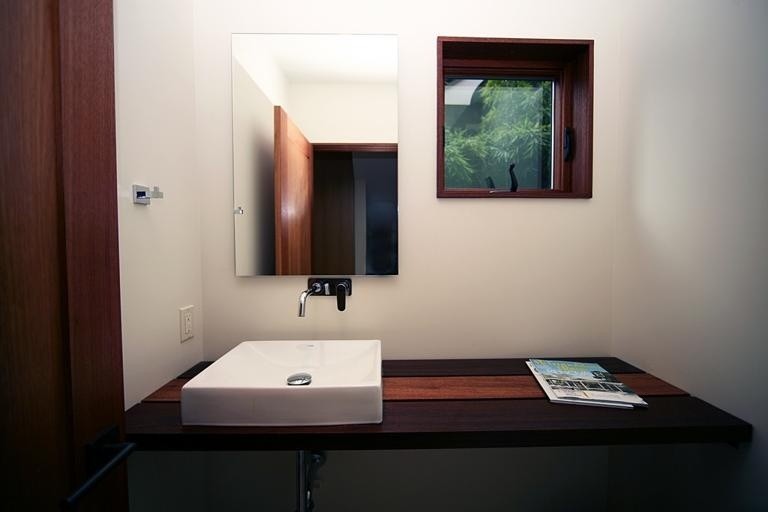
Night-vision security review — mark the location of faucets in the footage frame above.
[295,290,314,319]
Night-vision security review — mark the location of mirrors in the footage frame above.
[231,32,401,277]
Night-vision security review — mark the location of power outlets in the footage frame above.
[179,305,193,343]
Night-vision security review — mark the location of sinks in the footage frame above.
[181,339,383,427]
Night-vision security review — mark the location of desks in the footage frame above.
[125,358,753,512]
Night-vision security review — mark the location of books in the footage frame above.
[524,357,648,412]
